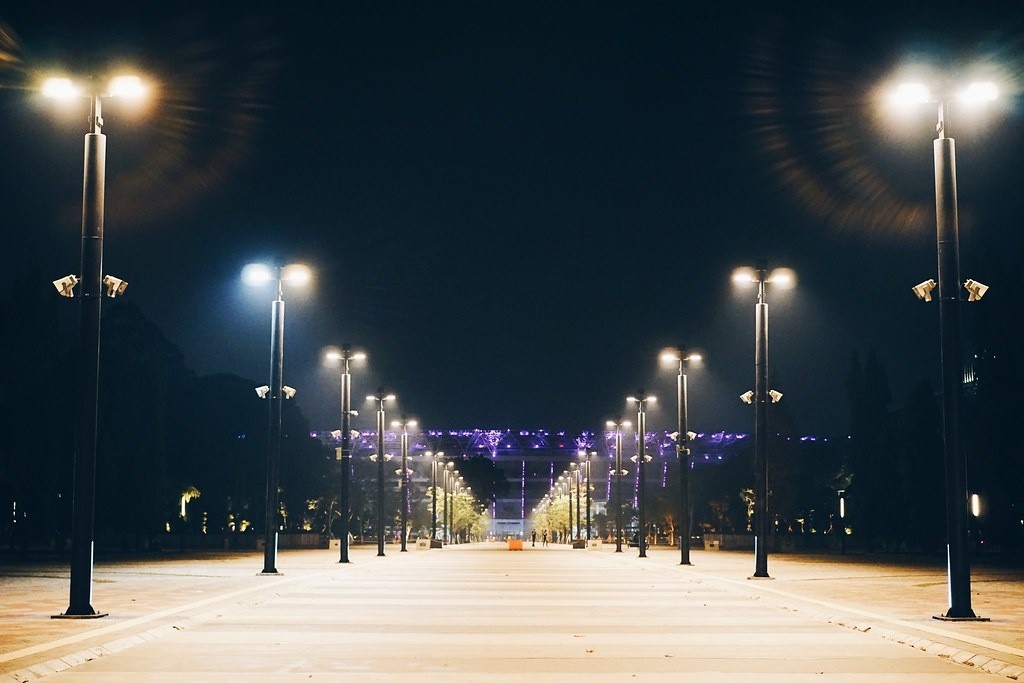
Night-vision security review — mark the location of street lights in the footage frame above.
[41,64,158,621]
[532,470,573,541]
[323,344,367,564]
[437,459,454,544]
[874,60,1013,624]
[577,449,597,539]
[391,415,418,552]
[365,388,396,556]
[424,451,445,539]
[838,489,847,555]
[729,259,802,579]
[447,469,485,544]
[243,258,316,575]
[606,416,632,552]
[970,492,982,560]
[625,389,658,558]
[571,461,586,539]
[656,345,705,566]
[180,495,186,553]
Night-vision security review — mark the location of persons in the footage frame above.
[542,530,548,546]
[531,529,536,547]
